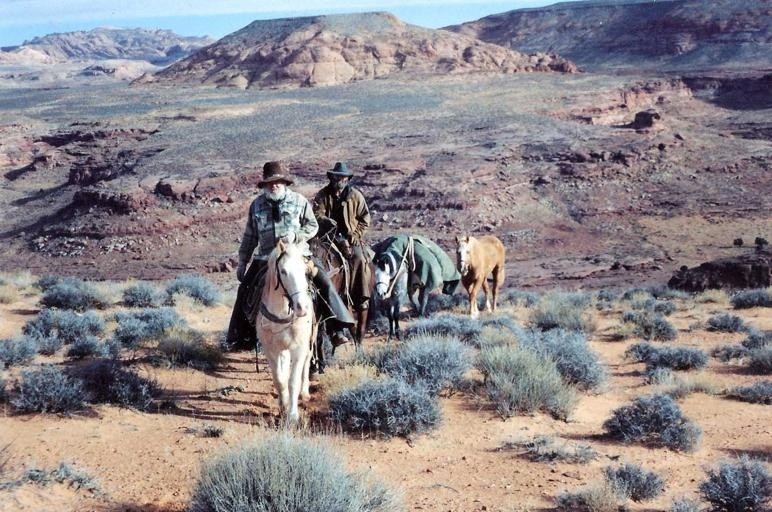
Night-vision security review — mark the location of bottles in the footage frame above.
[338,232,352,258]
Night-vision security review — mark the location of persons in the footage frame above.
[310,161,371,310]
[236,160,349,346]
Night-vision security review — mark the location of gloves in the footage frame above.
[346,231,361,247]
[279,231,295,243]
[324,218,337,228]
[237,261,247,282]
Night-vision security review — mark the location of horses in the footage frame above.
[373,251,408,343]
[455,234,506,319]
[255,236,315,427]
[307,234,375,356]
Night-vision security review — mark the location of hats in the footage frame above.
[326,162,353,182]
[257,160,294,189]
